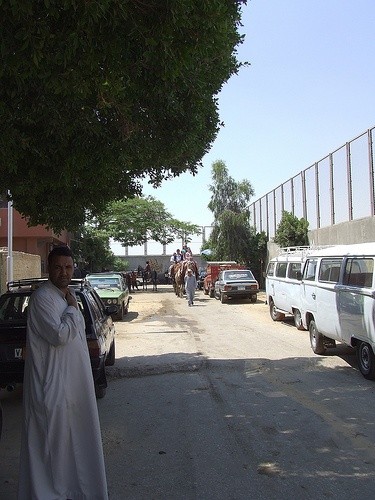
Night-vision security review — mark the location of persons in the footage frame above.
[124,243,199,307]
[15,245,109,500]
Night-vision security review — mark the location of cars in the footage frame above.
[134,269,152,286]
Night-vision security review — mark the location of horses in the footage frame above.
[137,265,158,291]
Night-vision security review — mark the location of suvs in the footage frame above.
[196,262,249,299]
[0,277,115,399]
[213,270,260,304]
[85,272,132,320]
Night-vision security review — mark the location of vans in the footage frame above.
[262,246,312,331]
[296,242,375,381]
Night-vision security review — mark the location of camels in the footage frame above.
[170,261,199,298]
